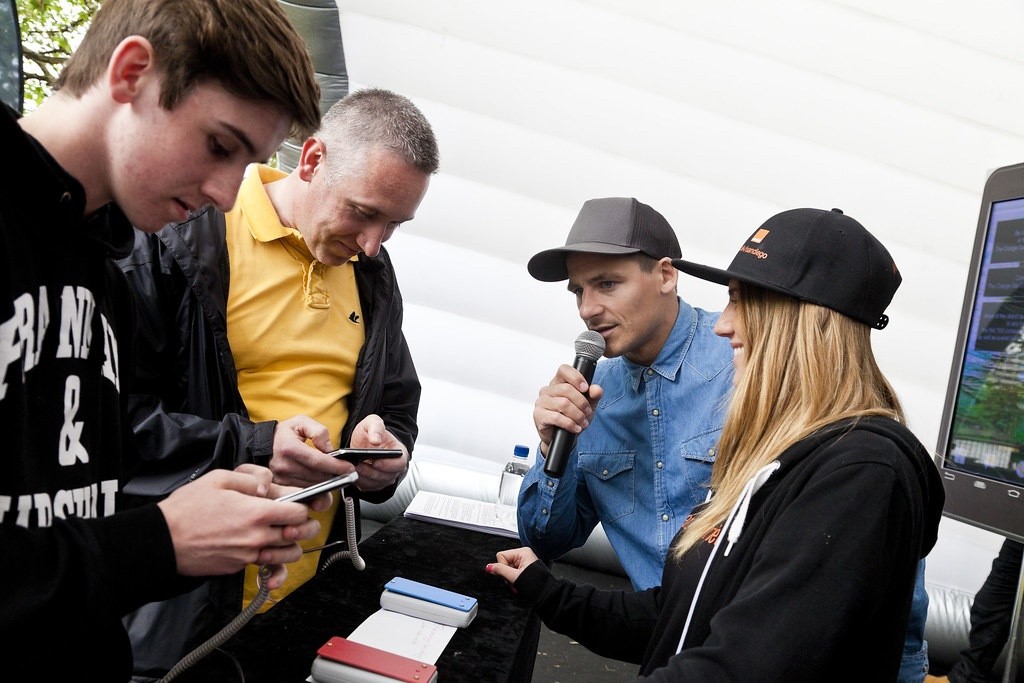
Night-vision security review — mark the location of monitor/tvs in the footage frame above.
[936,162,1024,542]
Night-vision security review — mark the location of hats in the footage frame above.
[527,197,682,282]
[671,208,902,330]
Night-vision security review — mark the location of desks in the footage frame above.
[167,514,542,683]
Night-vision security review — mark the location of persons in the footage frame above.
[485,206,945,683]
[118,88,441,683]
[0,0,335,683]
[925,539,1024,683]
[516,197,930,683]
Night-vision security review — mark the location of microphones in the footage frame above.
[543,331,605,479]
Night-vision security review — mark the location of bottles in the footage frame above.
[492,444,531,523]
